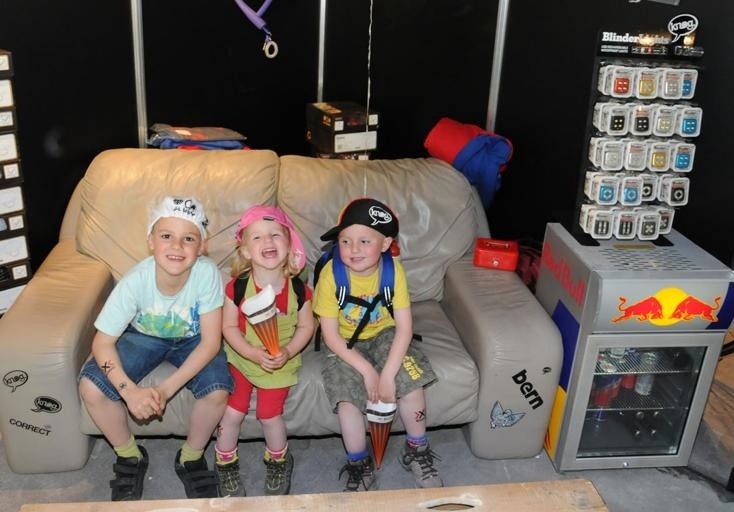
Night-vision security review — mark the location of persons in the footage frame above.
[313,198,444,493]
[77,198,234,501]
[214,206,314,498]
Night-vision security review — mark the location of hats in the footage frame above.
[235,205,306,271]
[147,196,209,240]
[320,197,399,239]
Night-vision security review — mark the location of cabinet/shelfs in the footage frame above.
[530,222,733,474]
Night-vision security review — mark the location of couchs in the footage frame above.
[0,148,563,474]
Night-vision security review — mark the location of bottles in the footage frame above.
[592,363,617,408]
[631,411,645,422]
[610,359,626,398]
[641,422,658,438]
[621,352,642,390]
[624,421,643,440]
[592,354,608,397]
[634,352,659,396]
[648,410,660,420]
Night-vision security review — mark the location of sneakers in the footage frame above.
[398,447,444,488]
[264,452,293,494]
[109,445,149,501]
[339,456,380,492]
[213,460,247,497]
[174,450,217,497]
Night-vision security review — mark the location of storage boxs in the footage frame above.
[473,233,518,271]
